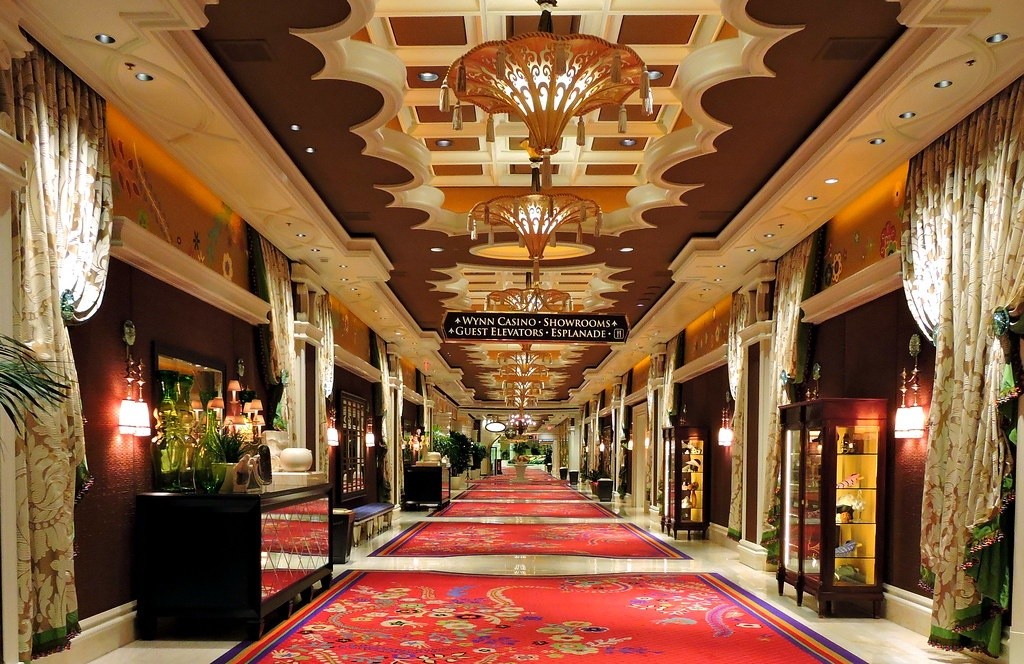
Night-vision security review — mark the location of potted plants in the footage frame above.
[581,468,608,494]
[781,370,807,401]
[435,433,491,490]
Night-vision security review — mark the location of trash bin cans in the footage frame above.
[333,508,356,563]
[598,478,613,501]
[547,463,552,472]
[569,470,579,485]
[559,467,568,479]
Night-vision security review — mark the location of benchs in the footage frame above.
[344,502,395,548]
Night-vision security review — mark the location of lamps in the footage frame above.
[586,430,634,453]
[437,0,658,193]
[328,407,339,447]
[717,407,733,446]
[893,370,924,440]
[485,288,575,419]
[366,416,375,446]
[118,322,151,437]
[469,159,602,279]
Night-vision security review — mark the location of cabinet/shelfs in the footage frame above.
[775,396,890,618]
[659,427,711,540]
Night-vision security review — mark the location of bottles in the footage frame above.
[404,436,427,465]
[155,370,227,495]
